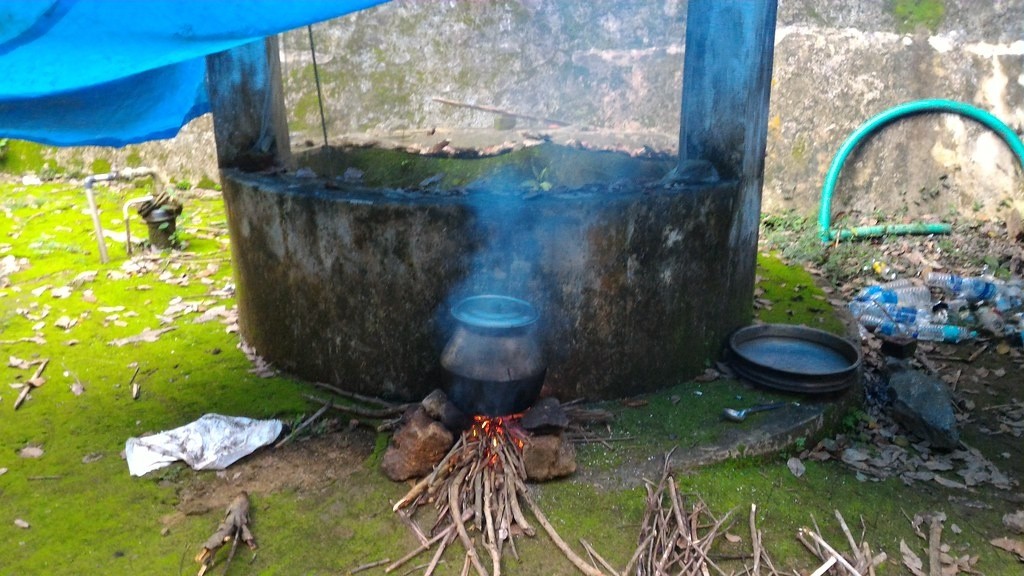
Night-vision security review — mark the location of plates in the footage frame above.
[730,323,861,391]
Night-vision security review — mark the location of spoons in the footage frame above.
[722,401,788,422]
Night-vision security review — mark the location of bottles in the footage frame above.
[846,271,1024,342]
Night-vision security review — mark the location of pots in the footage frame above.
[439,294,548,416]
